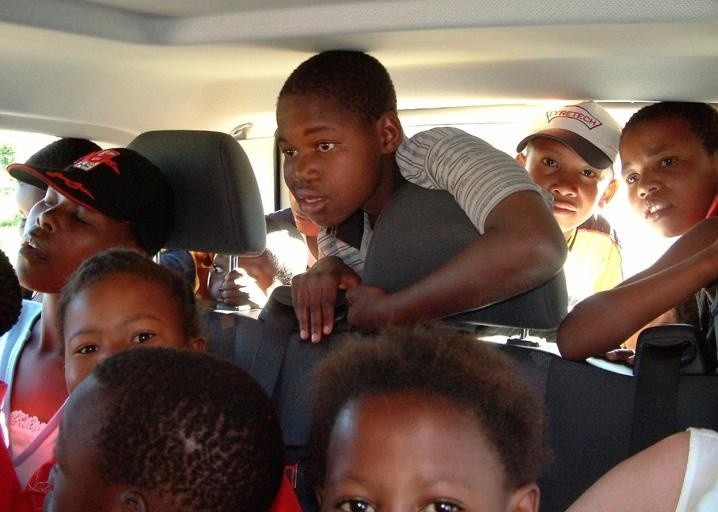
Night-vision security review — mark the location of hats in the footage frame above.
[5,147,178,250]
[515,99,624,169]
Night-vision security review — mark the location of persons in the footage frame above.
[1,137,309,511]
[276,51,567,344]
[557,100,718,358]
[507,99,629,356]
[294,338,718,512]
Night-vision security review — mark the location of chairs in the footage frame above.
[122,130,293,402]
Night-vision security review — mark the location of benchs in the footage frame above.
[280,181,718,512]
